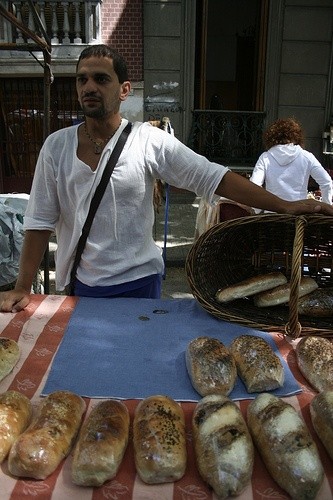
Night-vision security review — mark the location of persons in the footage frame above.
[0,44,333,314]
[248,118,333,215]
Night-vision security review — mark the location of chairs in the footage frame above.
[210,94,247,158]
[220,203,250,222]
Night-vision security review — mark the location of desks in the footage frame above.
[0,293,333,500]
[227,162,257,177]
[194,195,250,239]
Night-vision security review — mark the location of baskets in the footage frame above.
[186,213,333,339]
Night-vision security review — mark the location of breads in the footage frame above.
[133,395,187,484]
[71,399,130,486]
[247,393,324,500]
[0,337,21,382]
[7,391,86,480]
[310,390,333,466]
[228,334,285,393]
[185,337,238,397]
[192,394,254,498]
[215,271,287,303]
[295,336,333,392]
[0,390,33,466]
[298,288,333,317]
[254,277,319,307]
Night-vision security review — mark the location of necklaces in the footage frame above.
[84,116,122,154]
